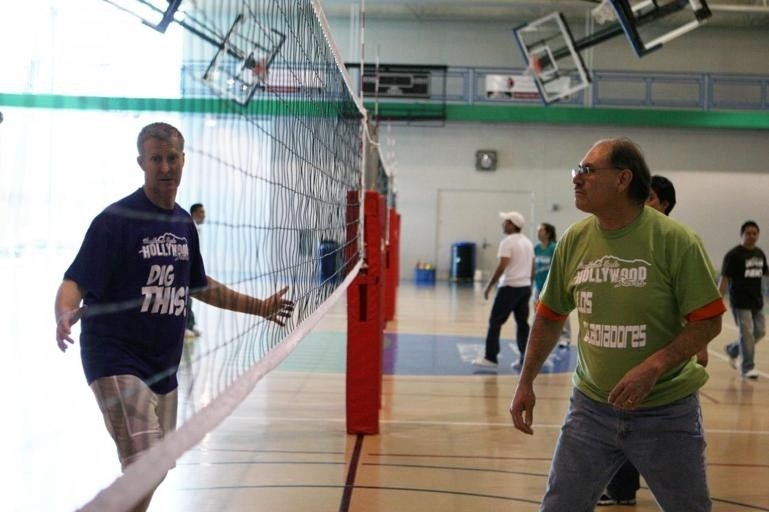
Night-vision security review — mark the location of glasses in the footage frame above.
[571,166,622,174]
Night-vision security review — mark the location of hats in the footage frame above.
[499,211,525,230]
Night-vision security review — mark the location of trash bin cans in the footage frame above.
[318,240,336,285]
[449,241,477,284]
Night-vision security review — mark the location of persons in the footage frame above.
[473,211,539,369]
[182,201,208,340]
[533,220,570,347]
[53,120,295,512]
[597,173,710,506]
[718,216,769,380]
[505,135,728,510]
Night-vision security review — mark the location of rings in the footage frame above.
[627,398,635,404]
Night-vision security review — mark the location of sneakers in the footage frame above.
[597,490,637,505]
[472,359,499,367]
[725,346,739,370]
[558,341,570,347]
[512,361,522,366]
[745,371,759,378]
